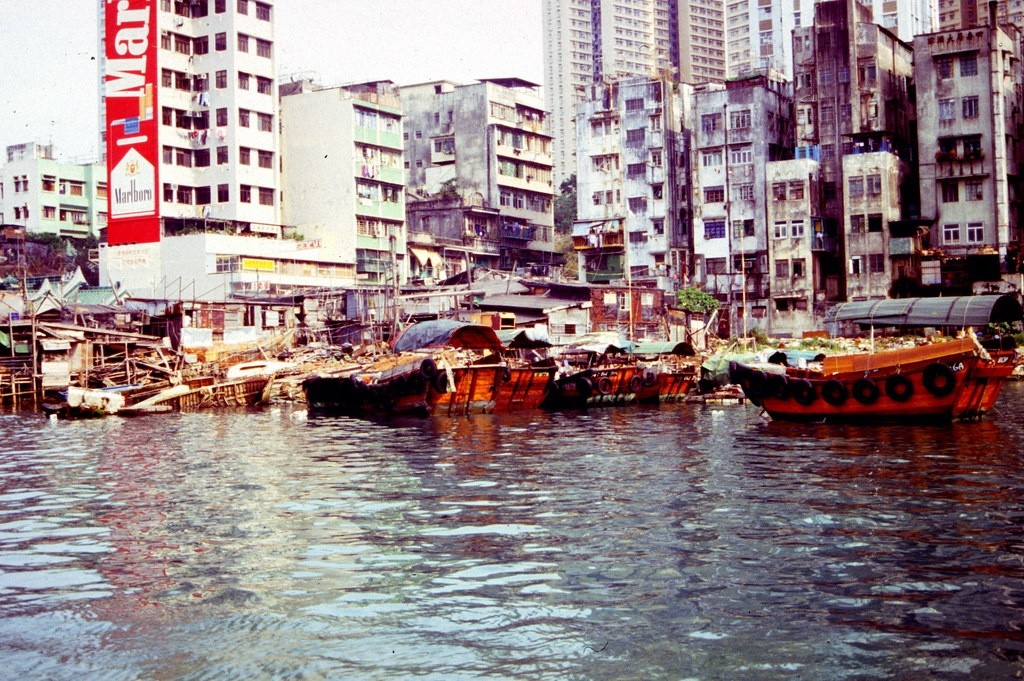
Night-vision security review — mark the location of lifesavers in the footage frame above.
[820,379,849,408]
[852,377,879,405]
[576,377,593,395]
[419,360,436,379]
[752,370,768,397]
[503,369,512,382]
[922,362,957,397]
[885,374,914,402]
[550,382,559,394]
[597,376,613,395]
[434,372,448,394]
[642,368,657,386]
[629,375,643,393]
[768,374,790,399]
[792,378,817,406]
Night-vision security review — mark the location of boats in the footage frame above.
[1,209,1023,421]
